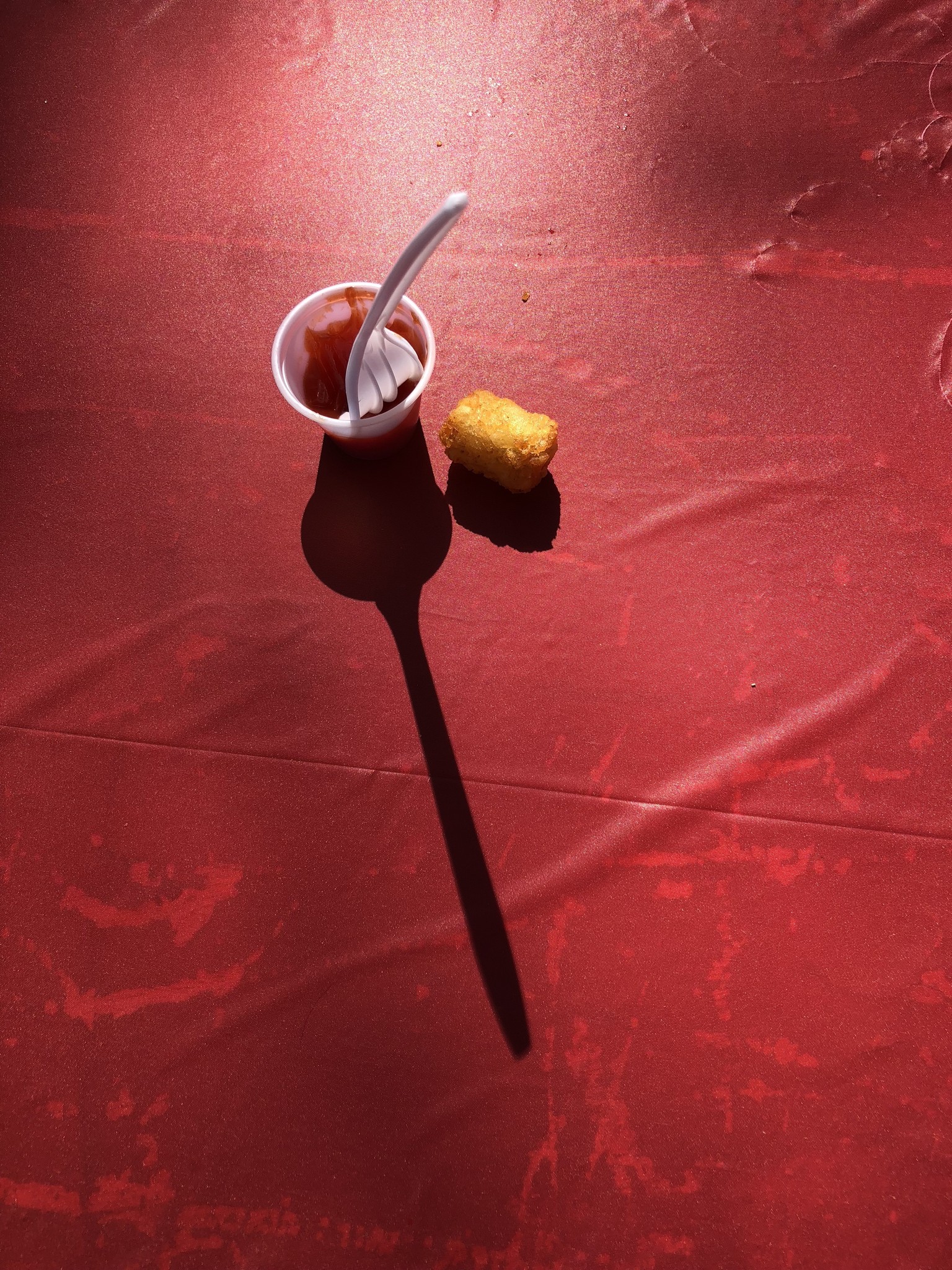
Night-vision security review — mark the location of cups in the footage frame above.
[272,282,437,460]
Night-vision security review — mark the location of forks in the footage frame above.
[335,191,471,423]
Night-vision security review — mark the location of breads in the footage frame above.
[438,390,559,492]
